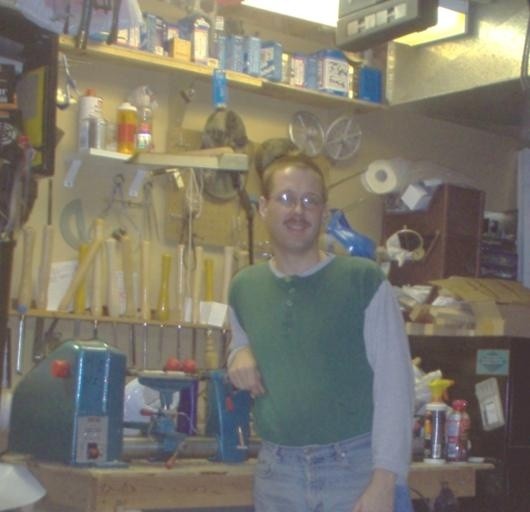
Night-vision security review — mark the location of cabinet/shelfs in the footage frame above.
[382,183,486,322]
[57,32,384,177]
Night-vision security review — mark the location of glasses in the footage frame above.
[262,189,325,209]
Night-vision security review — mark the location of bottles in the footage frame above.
[445,400,472,463]
[78,85,155,157]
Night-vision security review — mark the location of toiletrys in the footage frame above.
[249,30,262,75]
[229,29,244,72]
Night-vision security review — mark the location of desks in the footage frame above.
[2,454,501,512]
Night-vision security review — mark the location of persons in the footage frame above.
[224,152,418,511]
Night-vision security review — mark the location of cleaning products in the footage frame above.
[78,88,103,118]
[117,98,138,155]
[424,377,455,462]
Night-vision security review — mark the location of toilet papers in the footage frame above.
[360,157,414,196]
